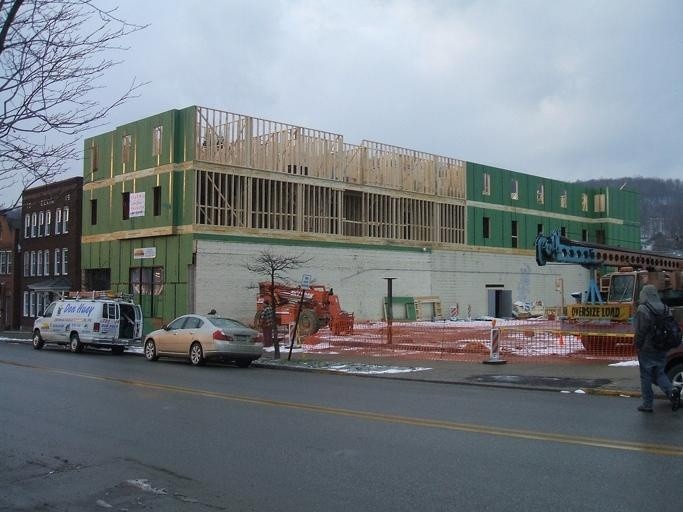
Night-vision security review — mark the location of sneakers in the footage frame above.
[672,386,680,410]
[638,405,652,411]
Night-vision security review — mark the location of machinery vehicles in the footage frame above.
[256,281,354,336]
[535,233,682,350]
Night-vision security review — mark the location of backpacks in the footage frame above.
[641,302,681,349]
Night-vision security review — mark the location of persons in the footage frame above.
[632,284,680,413]
[257,300,274,347]
[207,309,217,315]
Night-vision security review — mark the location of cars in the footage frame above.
[664,323,683,401]
[143,315,264,365]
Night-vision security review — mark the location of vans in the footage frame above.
[32,299,143,353]
